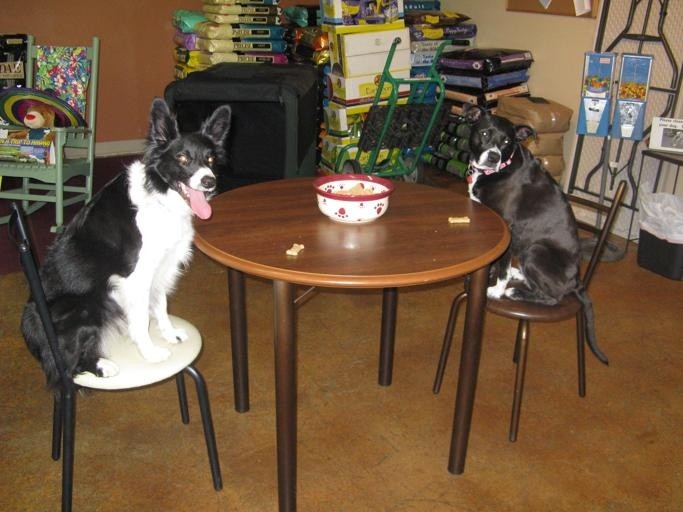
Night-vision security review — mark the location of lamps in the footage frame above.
[0,34,101,233]
[8,201,222,512]
[432,157,655,442]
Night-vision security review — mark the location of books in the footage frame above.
[317,1,413,174]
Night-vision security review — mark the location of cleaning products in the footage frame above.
[325,34,474,187]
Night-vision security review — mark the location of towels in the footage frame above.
[637,192,683,279]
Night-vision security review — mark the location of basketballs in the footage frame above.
[448,216,471,224]
[286,243,305,256]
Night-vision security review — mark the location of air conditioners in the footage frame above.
[19,97,233,399]
[461,101,611,367]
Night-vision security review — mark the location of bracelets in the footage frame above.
[310,173,396,225]
[314,222,387,254]
